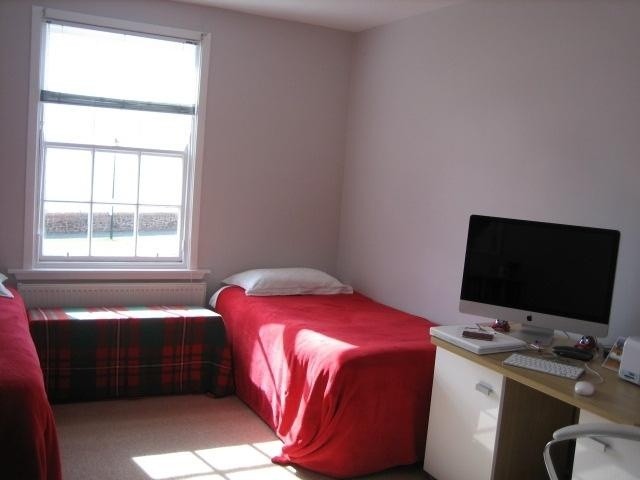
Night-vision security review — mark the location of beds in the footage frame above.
[0,271,61,480]
[208,267,450,476]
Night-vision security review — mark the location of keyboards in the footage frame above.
[503,352,585,382]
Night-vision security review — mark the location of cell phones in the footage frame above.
[461,329,495,342]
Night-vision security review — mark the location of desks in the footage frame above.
[420,321,639,480]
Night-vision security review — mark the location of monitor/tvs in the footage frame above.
[457,214,622,348]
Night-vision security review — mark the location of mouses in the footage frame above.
[575,380,595,397]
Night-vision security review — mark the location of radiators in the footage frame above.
[12,283,207,306]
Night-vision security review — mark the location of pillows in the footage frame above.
[224,267,354,297]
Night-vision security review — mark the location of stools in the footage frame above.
[31,309,228,396]
